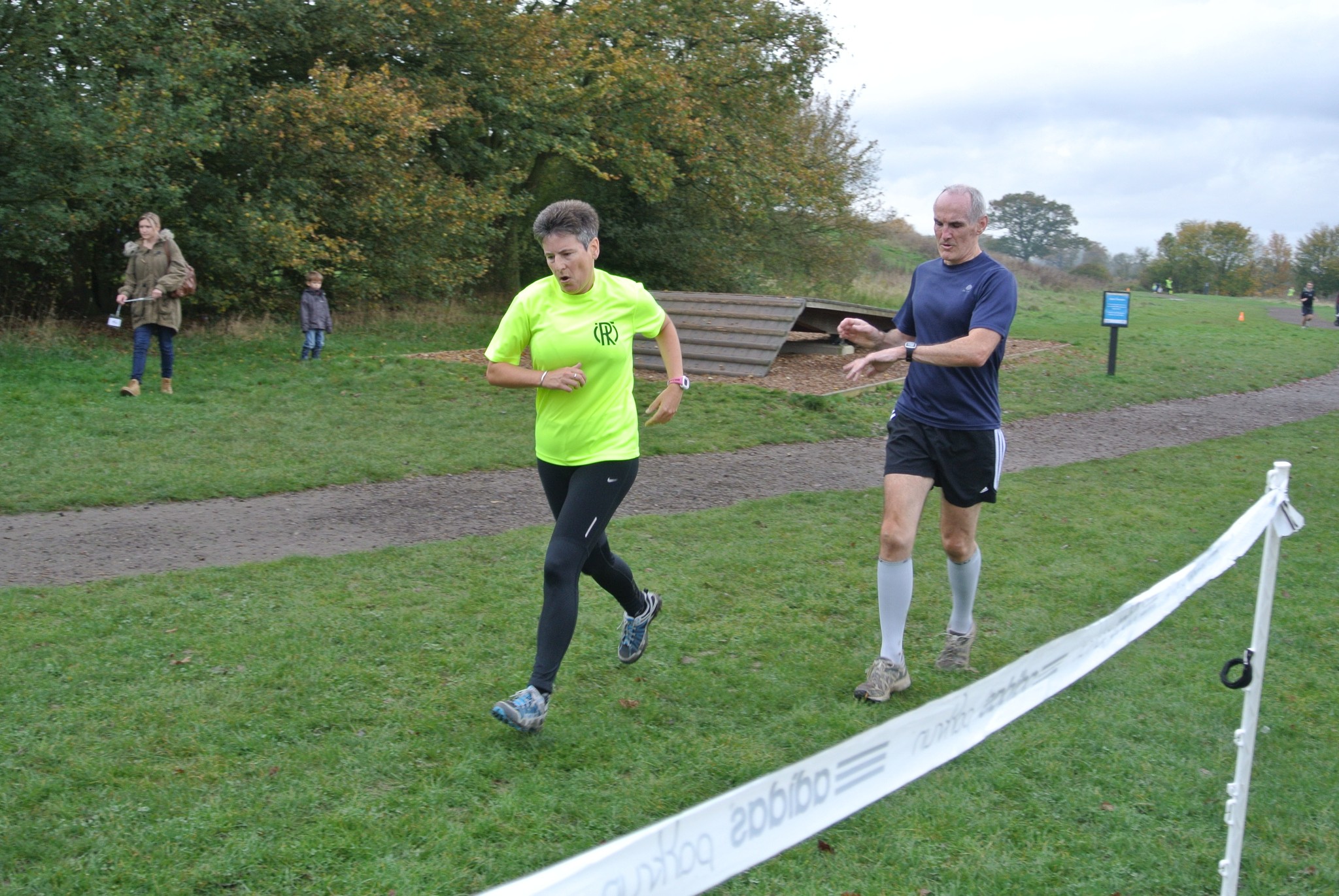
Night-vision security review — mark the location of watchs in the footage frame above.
[905,340,917,363]
[667,375,690,390]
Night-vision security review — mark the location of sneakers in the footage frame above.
[854,651,911,702]
[935,620,977,672]
[617,587,662,664]
[491,685,551,733]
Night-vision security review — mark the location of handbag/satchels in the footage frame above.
[164,241,197,299]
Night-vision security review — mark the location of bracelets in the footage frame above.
[539,369,548,388]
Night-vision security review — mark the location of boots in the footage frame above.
[120,379,141,397]
[161,378,173,394]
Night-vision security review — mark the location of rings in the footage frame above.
[574,373,577,380]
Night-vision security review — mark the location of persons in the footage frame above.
[834,185,1017,702]
[485,198,690,732]
[1152,282,1163,294]
[1287,286,1295,304]
[299,270,334,362]
[1334,289,1339,327]
[1165,276,1173,295]
[116,212,188,397]
[1299,281,1317,328]
